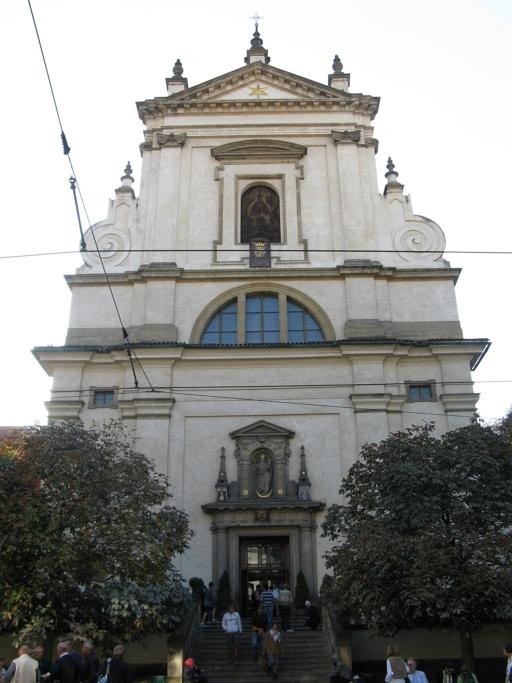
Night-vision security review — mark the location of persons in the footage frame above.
[383,644,410,682]
[253,452,274,496]
[222,604,243,664]
[501,642,512,682]
[406,658,429,682]
[436,662,458,683]
[201,582,219,628]
[261,622,283,680]
[328,654,350,682]
[249,603,269,661]
[96,645,132,682]
[248,582,294,631]
[301,599,320,632]
[182,657,202,682]
[1,637,101,682]
[455,665,478,682]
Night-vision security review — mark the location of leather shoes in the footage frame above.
[273,674,278,680]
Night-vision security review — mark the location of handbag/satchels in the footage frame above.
[97,657,112,682]
[258,654,268,670]
